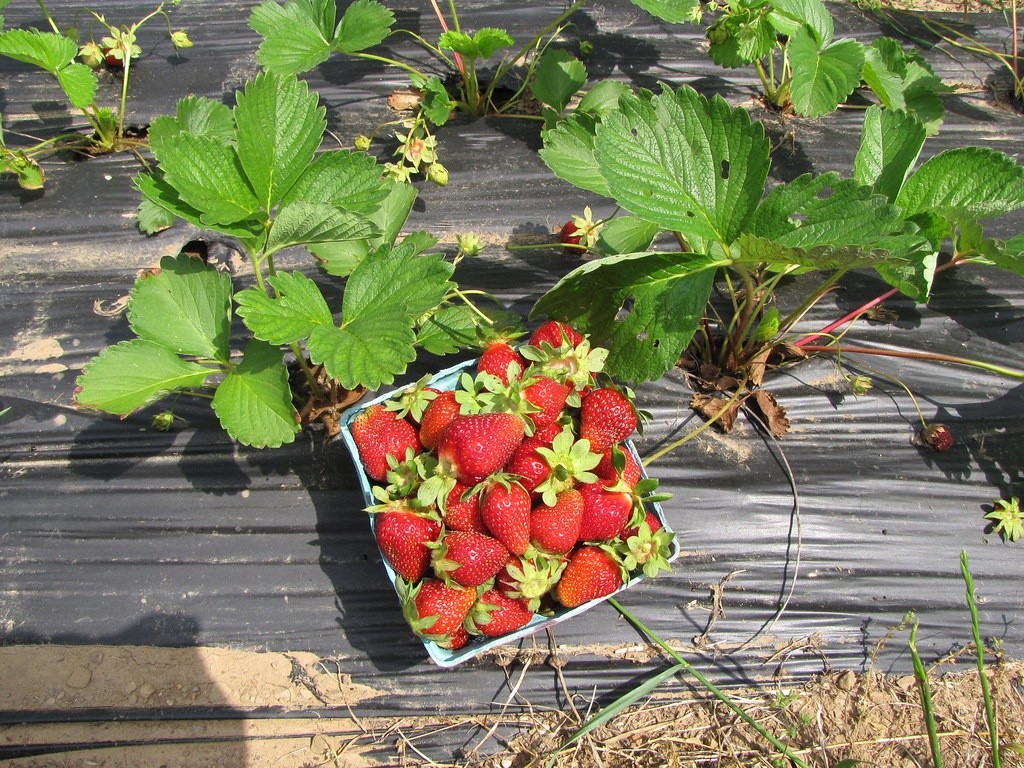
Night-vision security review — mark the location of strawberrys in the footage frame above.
[81,31,447,189]
[352,320,675,648]
[927,424,953,452]
[560,220,589,254]
